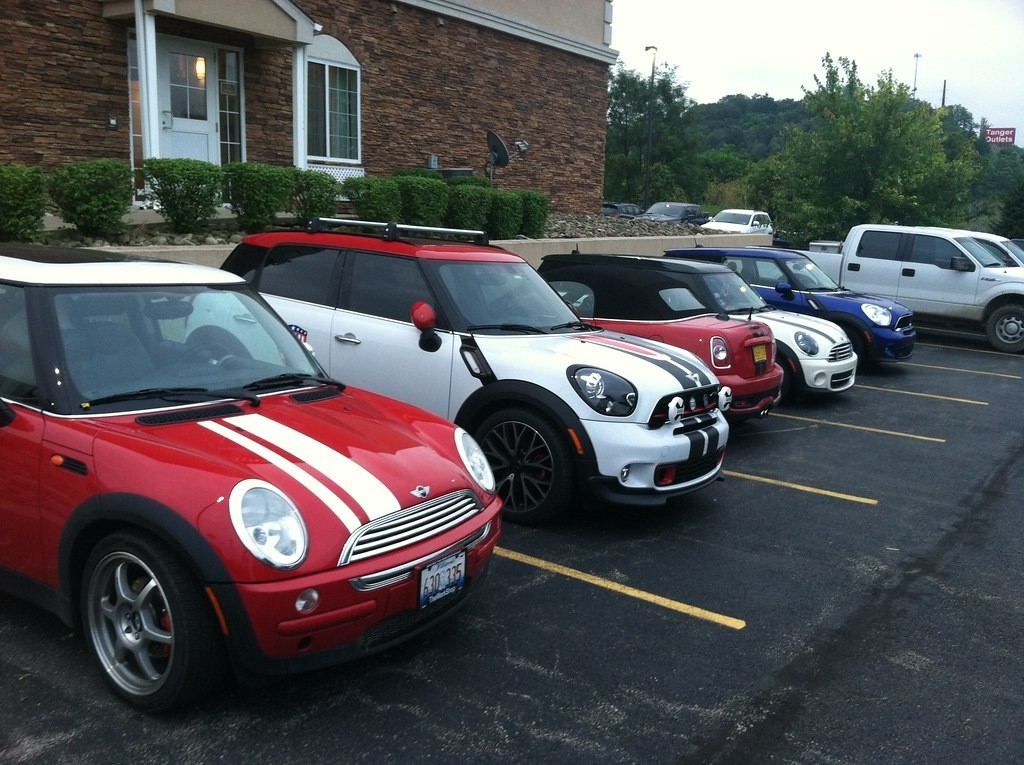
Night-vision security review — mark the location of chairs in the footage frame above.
[740,263,756,281]
[69,294,154,393]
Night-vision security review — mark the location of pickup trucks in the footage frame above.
[744,224,1024,355]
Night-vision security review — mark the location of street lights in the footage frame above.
[644,45,658,210]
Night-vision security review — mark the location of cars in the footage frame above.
[491,263,784,429]
[1,242,503,716]
[662,243,917,374]
[535,243,858,401]
[184,217,733,526]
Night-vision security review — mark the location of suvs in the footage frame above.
[634,202,710,228]
[602,200,646,222]
[701,208,775,237]
